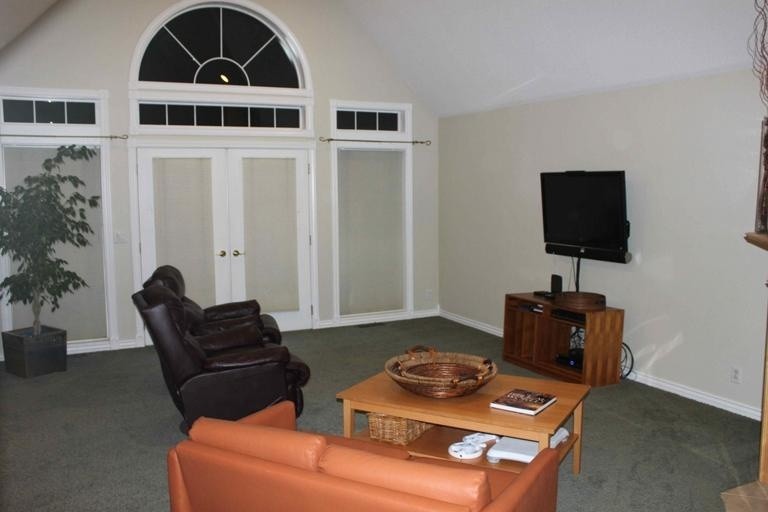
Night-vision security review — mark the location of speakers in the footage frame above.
[550,273,562,296]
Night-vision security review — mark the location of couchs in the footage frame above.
[167,400,559,511]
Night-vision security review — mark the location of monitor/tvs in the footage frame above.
[540,170,634,267]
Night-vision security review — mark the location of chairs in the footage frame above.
[132,284,311,434]
[143,265,282,345]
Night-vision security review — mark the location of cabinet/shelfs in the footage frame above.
[502,292,625,387]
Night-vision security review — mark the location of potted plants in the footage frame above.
[0,143,101,378]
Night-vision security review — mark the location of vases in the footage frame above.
[753,117,767,233]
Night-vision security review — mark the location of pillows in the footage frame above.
[187,415,326,471]
[318,442,490,512]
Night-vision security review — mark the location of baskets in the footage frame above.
[368,414,434,446]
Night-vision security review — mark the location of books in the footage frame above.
[489,388,558,415]
